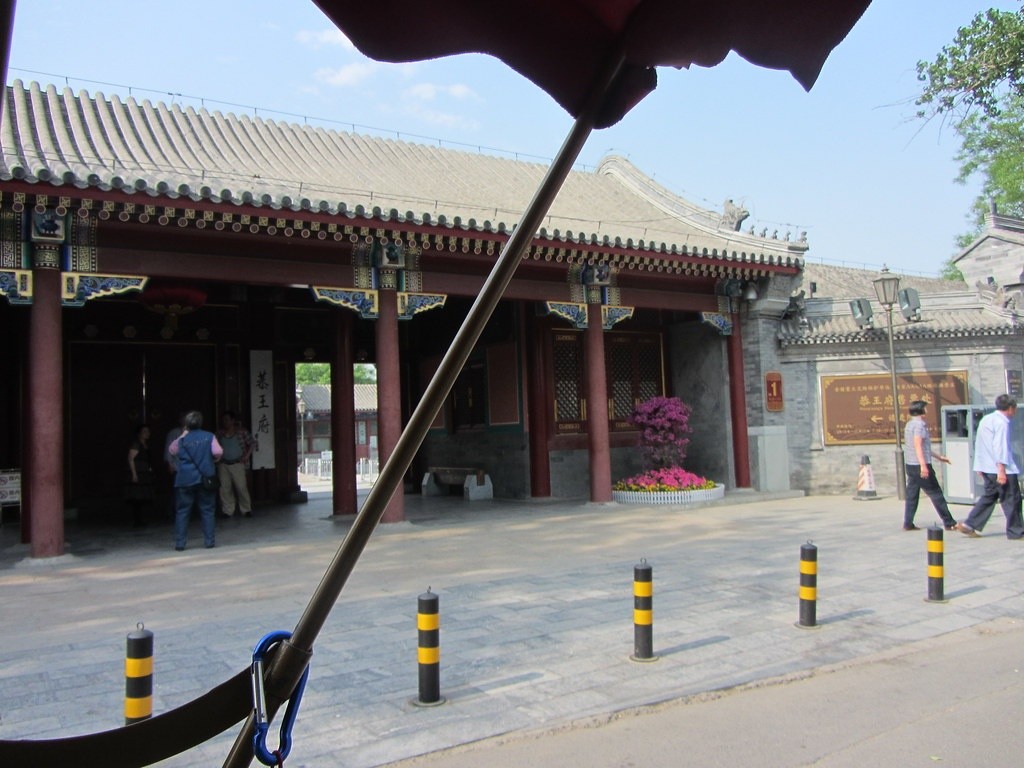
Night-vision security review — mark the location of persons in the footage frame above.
[902,400,958,530]
[127,424,168,528]
[168,411,226,551]
[163,413,191,534]
[958,393,1024,540]
[215,412,257,520]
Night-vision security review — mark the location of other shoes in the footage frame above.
[905,524,918,531]
[945,523,958,530]
[245,512,252,517]
[223,514,229,519]
[959,523,982,538]
[132,521,147,528]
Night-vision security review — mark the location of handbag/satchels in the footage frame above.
[203,475,221,490]
[143,465,162,485]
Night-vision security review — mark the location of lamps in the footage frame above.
[744,284,758,304]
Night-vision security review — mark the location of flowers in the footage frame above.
[613,397,716,491]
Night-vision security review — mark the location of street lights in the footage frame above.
[847,262,936,501]
[296,384,313,473]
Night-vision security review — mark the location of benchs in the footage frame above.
[422,467,494,500]
